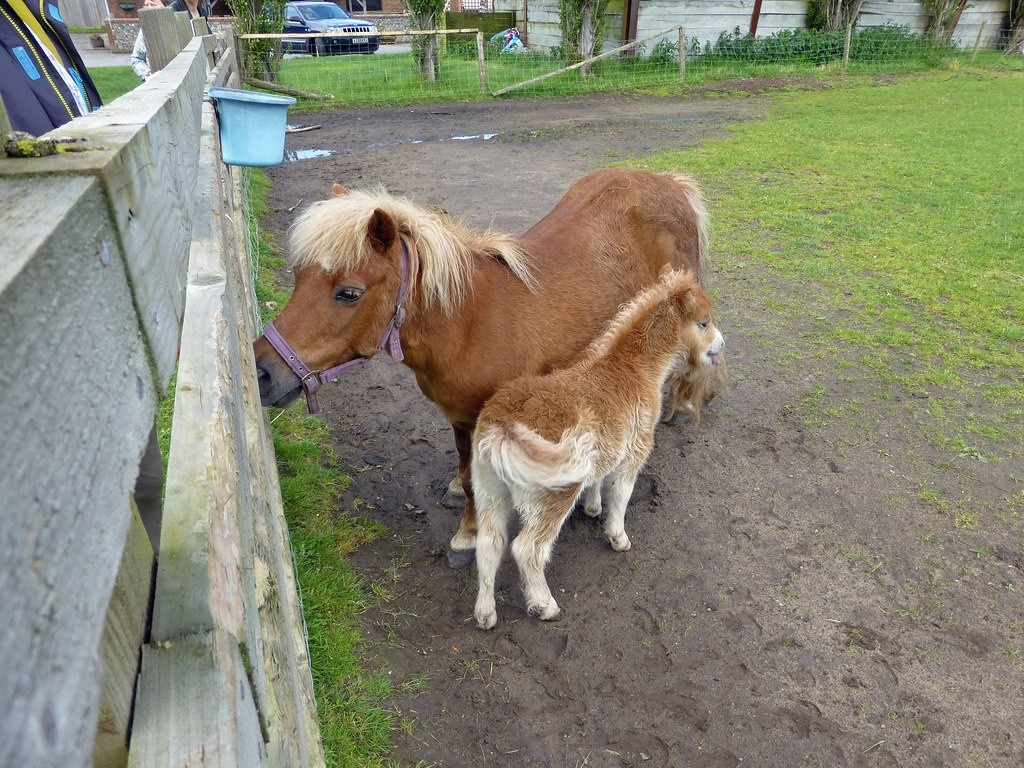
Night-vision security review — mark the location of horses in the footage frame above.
[251,164,727,631]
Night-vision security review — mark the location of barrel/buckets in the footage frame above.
[212,87,295,166]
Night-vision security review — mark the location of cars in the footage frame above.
[250,2,380,56]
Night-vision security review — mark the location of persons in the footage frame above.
[0,0,103,139]
[166,0,212,35]
[130,0,166,82]
